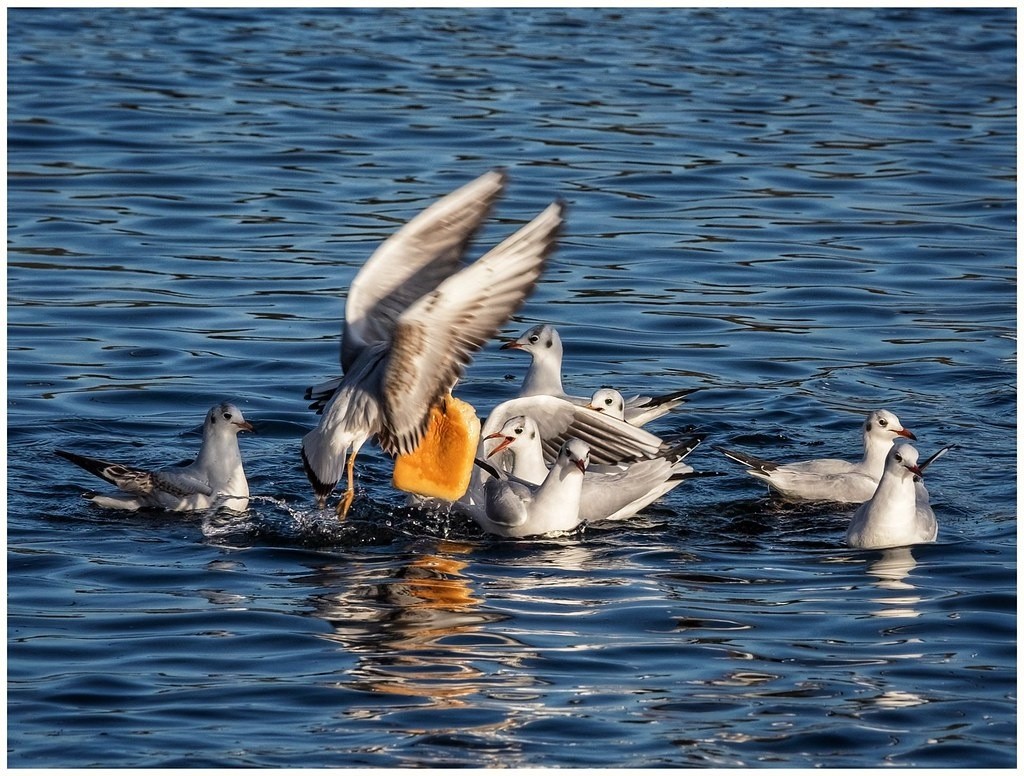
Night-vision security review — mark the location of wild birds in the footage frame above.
[298,170,566,519]
[468,323,704,541]
[722,408,951,548]
[55,402,259,516]
